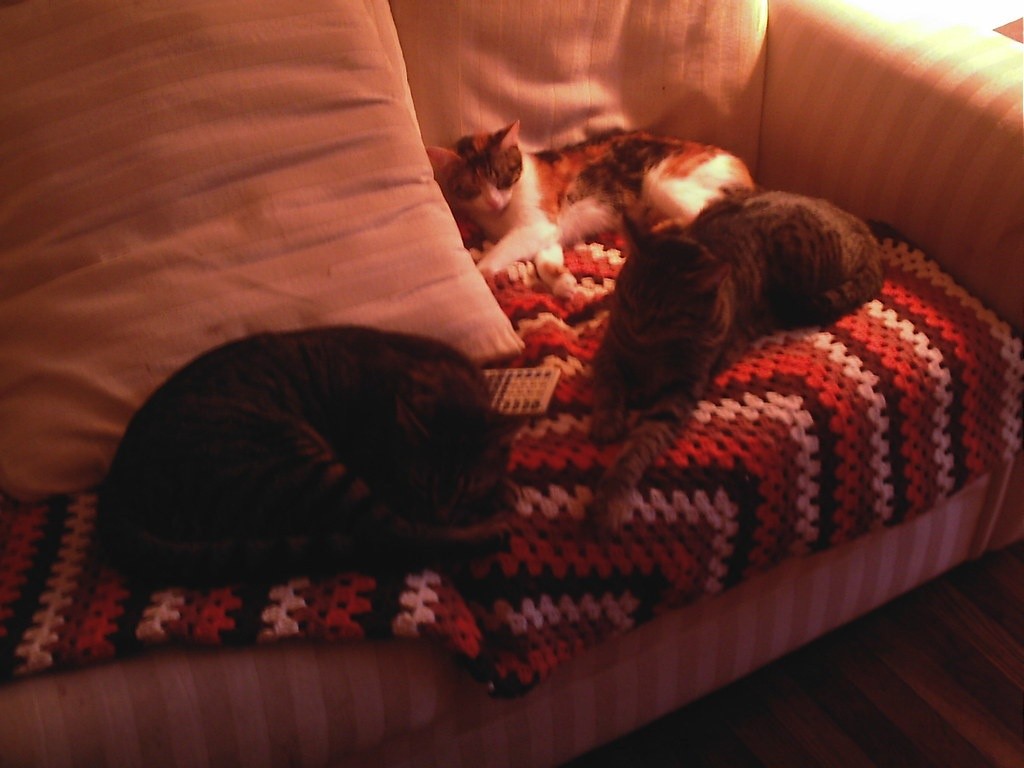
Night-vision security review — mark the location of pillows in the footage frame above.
[1,0,525,510]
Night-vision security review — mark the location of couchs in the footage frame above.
[0,0,1024,768]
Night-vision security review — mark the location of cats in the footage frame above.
[426,120,757,301]
[93,326,527,593]
[585,188,887,548]
